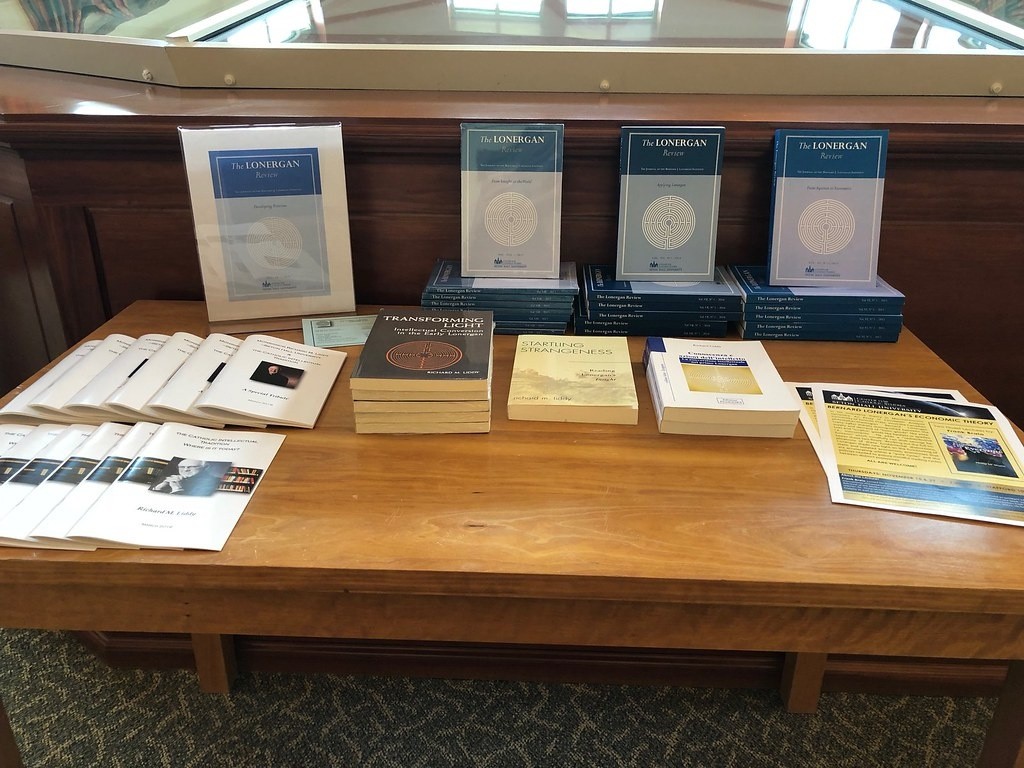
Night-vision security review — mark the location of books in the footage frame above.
[350,308,800,439]
[419,123,905,343]
[0,332,348,552]
[177,122,356,323]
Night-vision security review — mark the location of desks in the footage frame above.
[0,299,1024,715]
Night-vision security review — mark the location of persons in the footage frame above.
[251,365,288,386]
[153,459,221,496]
